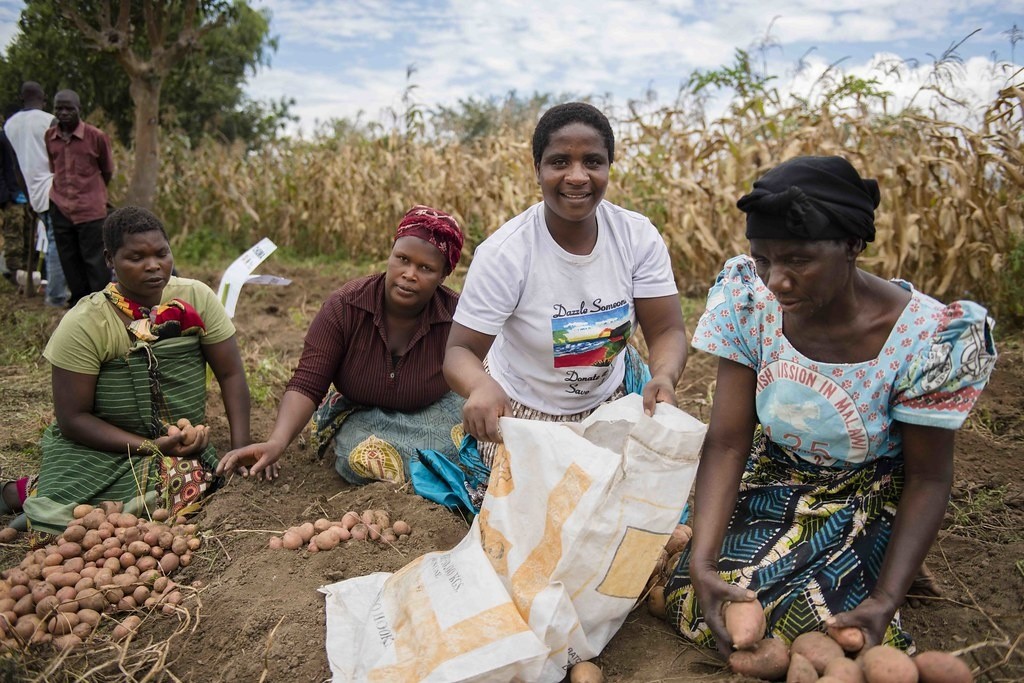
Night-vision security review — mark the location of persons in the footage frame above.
[441,101,688,471]
[217,205,467,487]
[664,156,997,663]
[0,80,115,315]
[0,207,282,542]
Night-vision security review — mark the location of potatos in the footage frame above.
[721,597,975,683]
[0,500,201,660]
[569,661,604,683]
[641,524,694,614]
[269,510,412,552]
[168,418,207,446]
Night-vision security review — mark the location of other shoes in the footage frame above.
[0,476,40,515]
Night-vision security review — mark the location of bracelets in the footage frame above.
[146,439,155,455]
